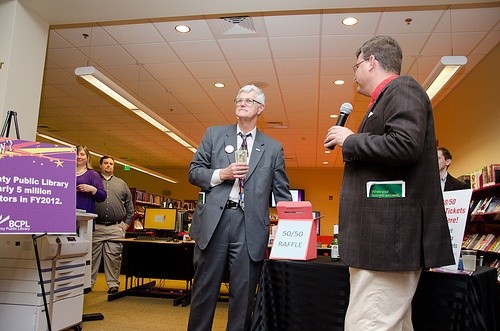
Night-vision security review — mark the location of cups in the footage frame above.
[461,249,478,272]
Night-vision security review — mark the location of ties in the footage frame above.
[238,133,252,200]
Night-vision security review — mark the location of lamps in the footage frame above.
[37,27,198,183]
[416,4,467,100]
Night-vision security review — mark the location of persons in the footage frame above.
[84,156,135,293]
[76,144,107,234]
[323,35,456,331]
[188,85,294,331]
[438,147,471,224]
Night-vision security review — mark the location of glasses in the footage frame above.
[233,97,261,106]
[352,57,378,72]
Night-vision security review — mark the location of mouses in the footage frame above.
[173,238,179,242]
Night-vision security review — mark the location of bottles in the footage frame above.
[330,224,341,262]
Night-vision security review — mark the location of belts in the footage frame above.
[95,220,124,226]
[225,201,240,209]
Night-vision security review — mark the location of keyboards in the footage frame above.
[133,236,172,242]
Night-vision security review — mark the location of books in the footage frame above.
[460,164,500,281]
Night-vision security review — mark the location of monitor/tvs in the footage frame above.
[270,188,305,208]
[143,207,177,237]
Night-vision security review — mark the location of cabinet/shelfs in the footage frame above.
[460,183,500,266]
[126,187,161,231]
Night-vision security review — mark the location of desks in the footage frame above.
[107,236,189,306]
[181,238,231,307]
[249,253,500,331]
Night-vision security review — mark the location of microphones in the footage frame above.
[327,103,353,150]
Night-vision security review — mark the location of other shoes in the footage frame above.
[107,287,118,294]
[84,288,91,294]
